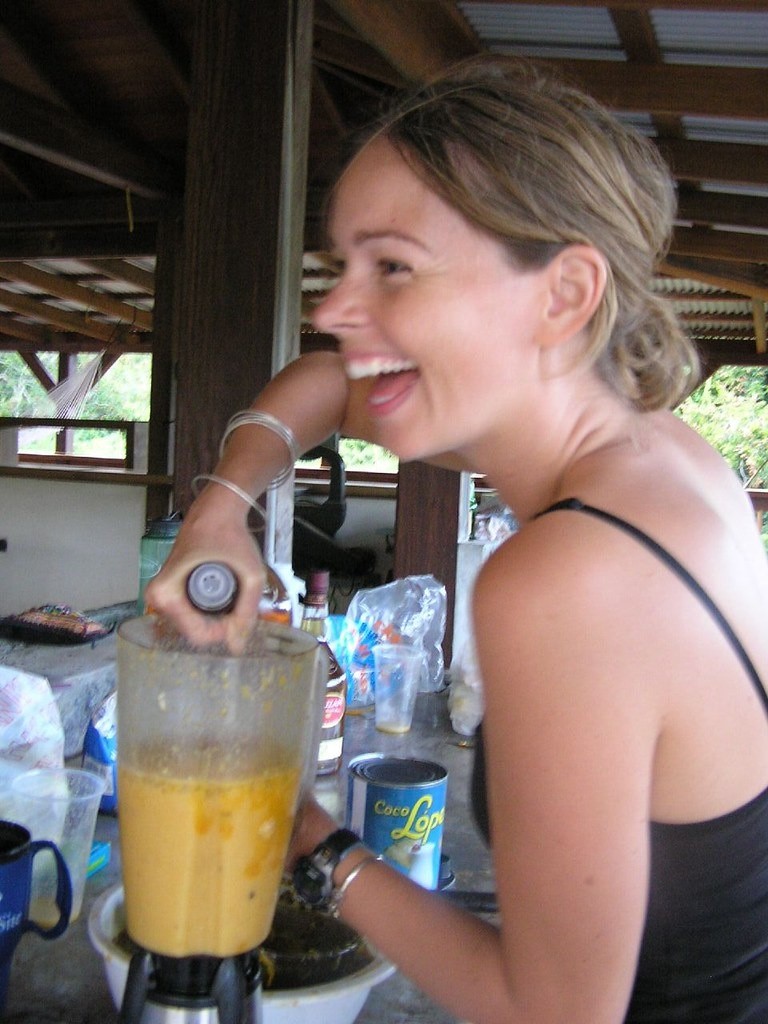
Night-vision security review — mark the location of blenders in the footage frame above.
[117,615,320,1024]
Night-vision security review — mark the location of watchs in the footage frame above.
[292,828,364,914]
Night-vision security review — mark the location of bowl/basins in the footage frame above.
[90,872,397,1024]
[328,613,408,706]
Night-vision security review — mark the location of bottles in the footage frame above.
[188,559,290,624]
[300,568,346,776]
[137,509,183,616]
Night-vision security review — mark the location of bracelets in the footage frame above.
[191,474,270,532]
[331,854,386,920]
[219,411,301,490]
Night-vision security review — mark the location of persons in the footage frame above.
[142,62,768,1024]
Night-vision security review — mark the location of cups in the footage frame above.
[0,817,73,999]
[12,768,106,927]
[372,644,423,732]
